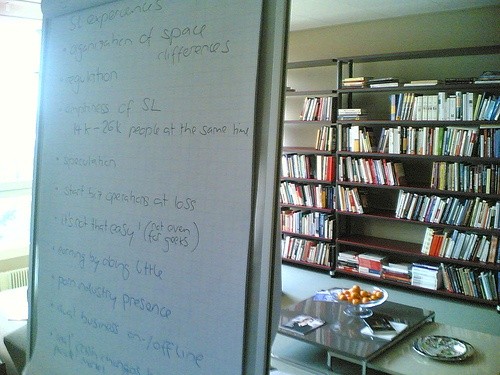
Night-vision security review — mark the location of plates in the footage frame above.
[412,335,475,361]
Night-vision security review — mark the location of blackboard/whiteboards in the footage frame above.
[22,0,290,375]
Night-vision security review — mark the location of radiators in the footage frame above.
[6,266,30,289]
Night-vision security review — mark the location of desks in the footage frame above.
[0,283,30,375]
[279,286,500,375]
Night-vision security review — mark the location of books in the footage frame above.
[362,313,396,333]
[280,76,500,302]
[278,314,328,335]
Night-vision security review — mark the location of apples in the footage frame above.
[338,285,382,304]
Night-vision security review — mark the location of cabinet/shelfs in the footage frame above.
[279,44,500,311]
